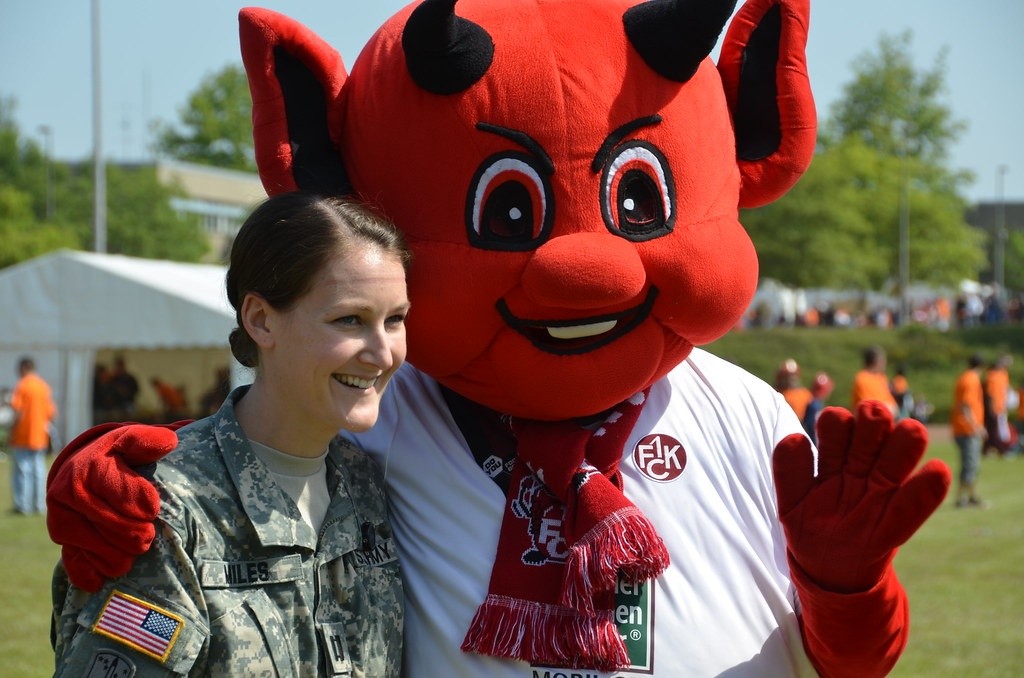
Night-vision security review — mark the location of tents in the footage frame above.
[0,246,257,454]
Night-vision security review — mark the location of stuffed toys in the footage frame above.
[46,0,950,678]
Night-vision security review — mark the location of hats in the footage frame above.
[866,347,887,365]
[778,359,801,376]
[812,375,834,396]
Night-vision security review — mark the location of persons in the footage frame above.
[7,357,56,517]
[50,191,410,677]
[753,282,1024,513]
[93,354,229,426]
